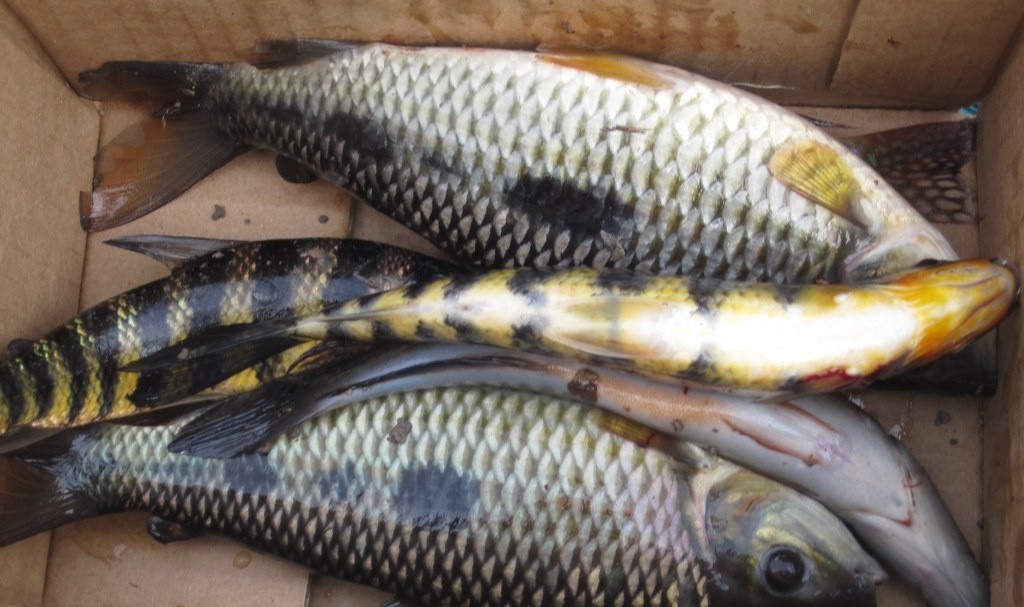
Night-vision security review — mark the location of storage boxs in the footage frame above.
[0,0,1024,607]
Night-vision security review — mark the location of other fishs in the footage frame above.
[0,38,1016,606]
